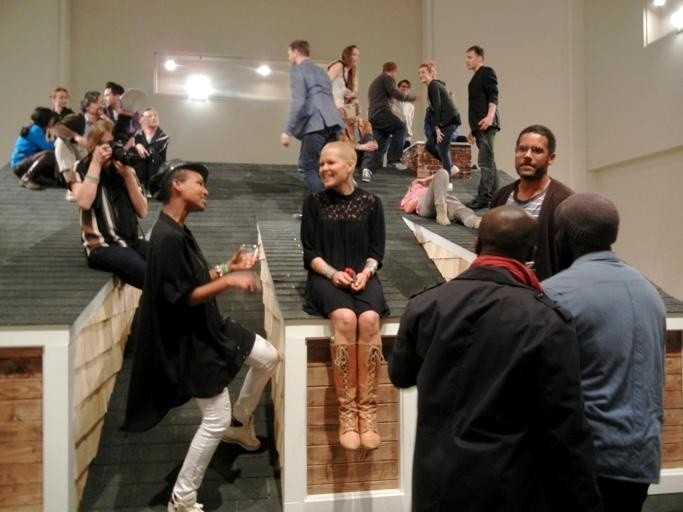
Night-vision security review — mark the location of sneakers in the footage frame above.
[168,491,204,512]
[451,165,459,177]
[392,160,407,170]
[362,168,372,181]
[222,415,260,451]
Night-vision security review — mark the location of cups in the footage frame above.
[238,243,257,265]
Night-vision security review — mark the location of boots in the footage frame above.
[330,343,360,450]
[436,203,451,226]
[359,343,382,450]
[18,159,40,189]
[462,213,482,229]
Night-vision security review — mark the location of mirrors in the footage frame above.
[150,50,328,104]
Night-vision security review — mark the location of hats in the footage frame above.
[149,158,209,200]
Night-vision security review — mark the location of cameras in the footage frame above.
[110,140,140,167]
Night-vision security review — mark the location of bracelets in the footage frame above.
[215,263,228,276]
[364,259,376,275]
[321,266,335,279]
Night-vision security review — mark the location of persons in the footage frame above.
[12,82,168,199]
[387,204,608,511]
[400,169,482,229]
[70,119,149,289]
[418,63,461,190]
[300,141,391,450]
[466,46,499,209]
[491,125,573,283]
[119,157,279,511]
[281,40,422,218]
[536,191,667,511]
